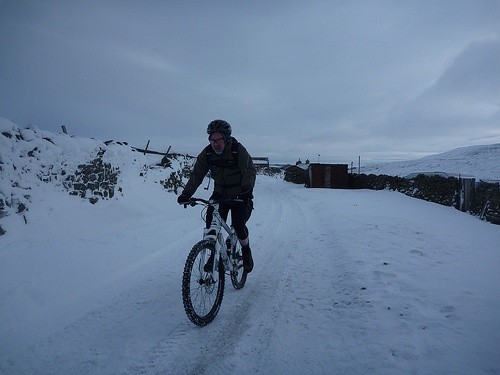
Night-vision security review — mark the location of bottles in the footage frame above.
[226,236,232,258]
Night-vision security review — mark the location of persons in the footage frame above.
[176,120,256,273]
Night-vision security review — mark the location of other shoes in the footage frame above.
[242,248,254,273]
[203,252,214,272]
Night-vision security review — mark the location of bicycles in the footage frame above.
[178,196,248,328]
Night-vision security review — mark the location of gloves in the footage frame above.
[177,190,192,208]
[238,188,254,202]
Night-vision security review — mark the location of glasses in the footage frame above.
[209,135,225,143]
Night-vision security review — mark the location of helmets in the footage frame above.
[206,120,231,135]
